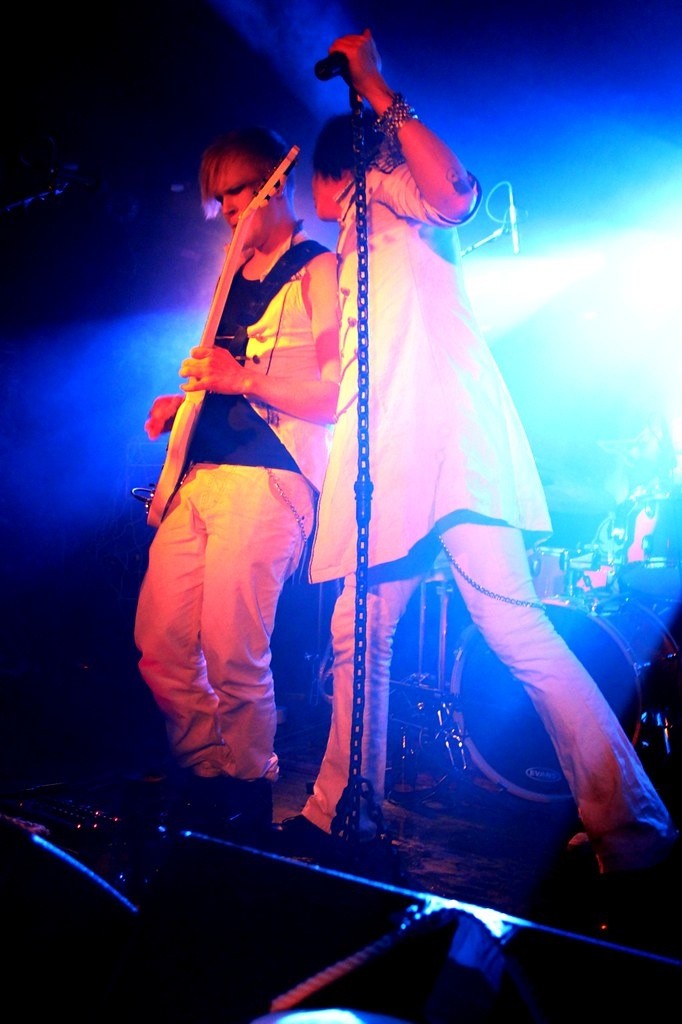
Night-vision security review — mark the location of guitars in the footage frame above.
[131,140,300,531]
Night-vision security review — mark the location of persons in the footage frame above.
[272,28,674,879]
[133,124,343,838]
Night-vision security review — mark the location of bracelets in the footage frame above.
[372,91,418,144]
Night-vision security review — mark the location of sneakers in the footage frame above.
[271,816,340,861]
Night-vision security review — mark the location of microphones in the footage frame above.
[314,51,347,80]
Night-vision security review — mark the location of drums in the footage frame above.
[451,592,681,804]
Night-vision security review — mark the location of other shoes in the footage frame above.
[193,769,275,851]
